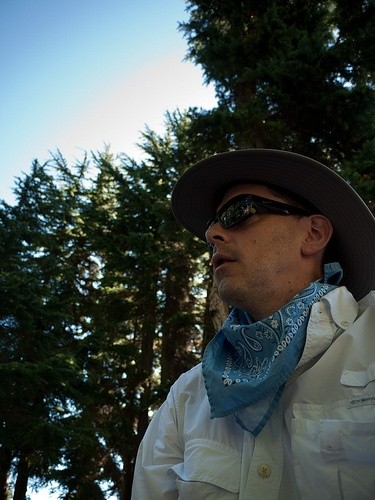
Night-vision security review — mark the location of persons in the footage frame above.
[131,148,375,500]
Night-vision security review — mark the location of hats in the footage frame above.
[172,149,375,300]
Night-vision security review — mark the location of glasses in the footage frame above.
[203,193,312,247]
